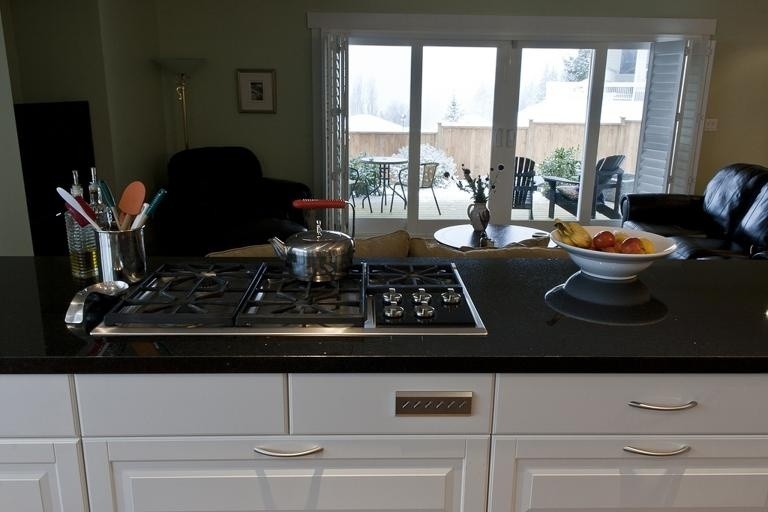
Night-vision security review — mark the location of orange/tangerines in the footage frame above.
[615,232,624,239]
[640,239,656,253]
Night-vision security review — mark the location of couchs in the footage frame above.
[620,163,767,259]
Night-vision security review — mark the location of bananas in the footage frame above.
[555,218,592,248]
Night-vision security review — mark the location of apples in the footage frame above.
[593,231,615,248]
[619,238,644,253]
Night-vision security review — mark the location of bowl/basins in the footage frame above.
[550,226,678,280]
[543,270,672,328]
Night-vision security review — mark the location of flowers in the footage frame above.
[443,163,505,202]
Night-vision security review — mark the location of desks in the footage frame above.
[358,157,408,209]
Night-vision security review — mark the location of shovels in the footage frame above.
[118,181,145,230]
[66,196,100,233]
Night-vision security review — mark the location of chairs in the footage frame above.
[512,157,538,219]
[543,155,625,220]
[348,166,372,213]
[151,147,312,256]
[389,163,441,215]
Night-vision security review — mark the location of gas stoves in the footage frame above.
[90,261,488,336]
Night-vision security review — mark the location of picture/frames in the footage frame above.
[236,68,277,114]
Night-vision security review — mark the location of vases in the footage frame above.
[467,203,490,232]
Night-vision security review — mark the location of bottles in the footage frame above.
[64,170,102,278]
[85,166,113,231]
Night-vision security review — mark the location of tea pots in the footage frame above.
[266,198,356,284]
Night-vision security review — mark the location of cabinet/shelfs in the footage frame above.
[1,357,89,512]
[486,359,768,512]
[71,358,495,511]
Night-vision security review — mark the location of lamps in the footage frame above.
[151,57,205,150]
